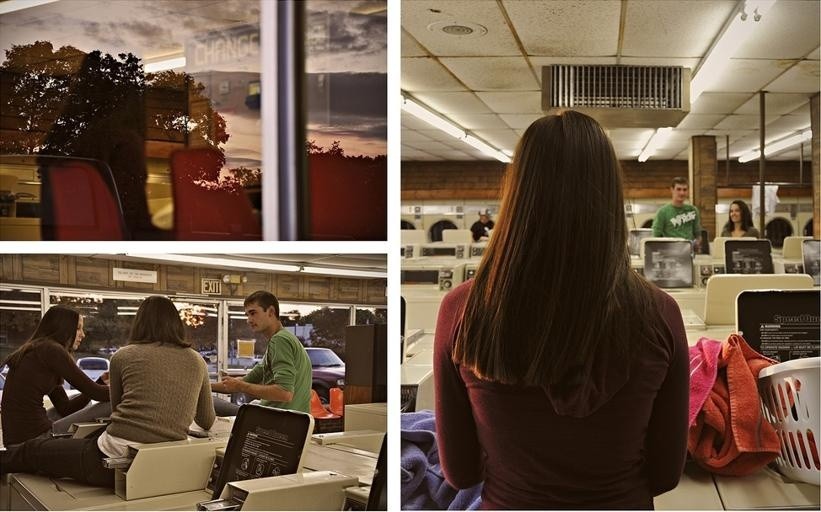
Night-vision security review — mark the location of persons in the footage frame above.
[0,296,217,488]
[433,112,689,510]
[471,208,495,241]
[211,291,313,417]
[721,201,759,239]
[0,305,110,450]
[37,50,175,241]
[652,177,702,254]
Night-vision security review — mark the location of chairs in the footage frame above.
[38,148,261,241]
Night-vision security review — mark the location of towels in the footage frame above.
[689,334,818,476]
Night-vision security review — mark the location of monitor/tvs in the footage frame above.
[640,236,821,288]
[211,404,315,499]
[736,290,821,363]
[630,229,652,255]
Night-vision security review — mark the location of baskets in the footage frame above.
[758,356,821,486]
